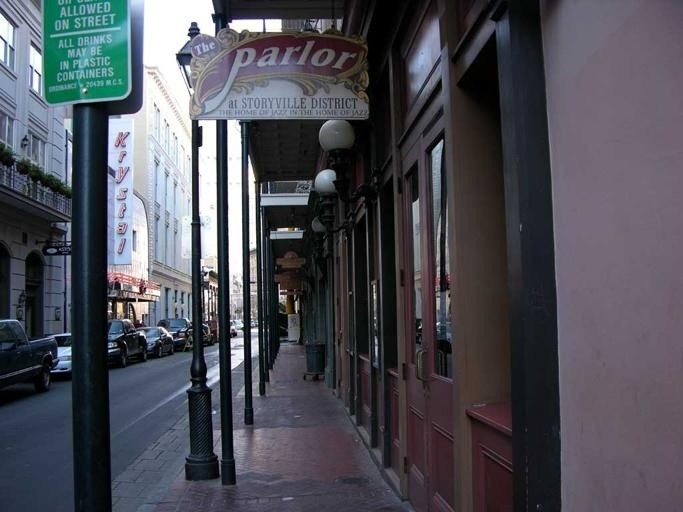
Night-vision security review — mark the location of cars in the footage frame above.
[50,320,260,380]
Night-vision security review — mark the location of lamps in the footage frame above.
[318,119,378,205]
[19,289,27,303]
[314,168,350,234]
[311,215,333,262]
[21,135,29,148]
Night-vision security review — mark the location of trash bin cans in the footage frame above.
[303,341,325,381]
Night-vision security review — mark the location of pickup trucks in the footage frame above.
[0,319,60,393]
